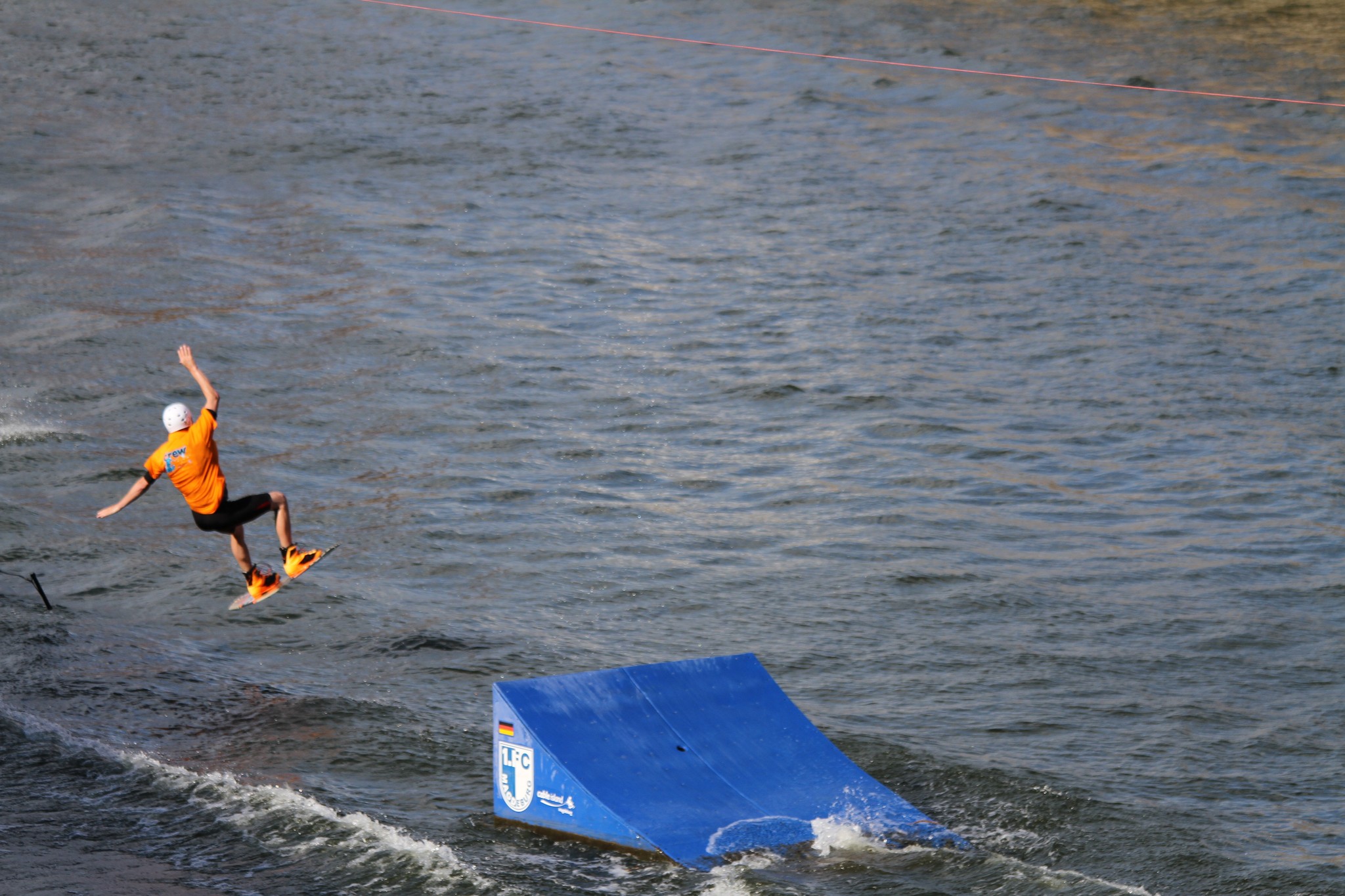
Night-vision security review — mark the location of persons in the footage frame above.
[94,343,321,600]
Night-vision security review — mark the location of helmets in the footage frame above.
[162,403,193,434]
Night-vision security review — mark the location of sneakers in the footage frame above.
[242,564,280,600]
[278,544,322,579]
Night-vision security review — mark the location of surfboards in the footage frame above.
[226,541,343,612]
